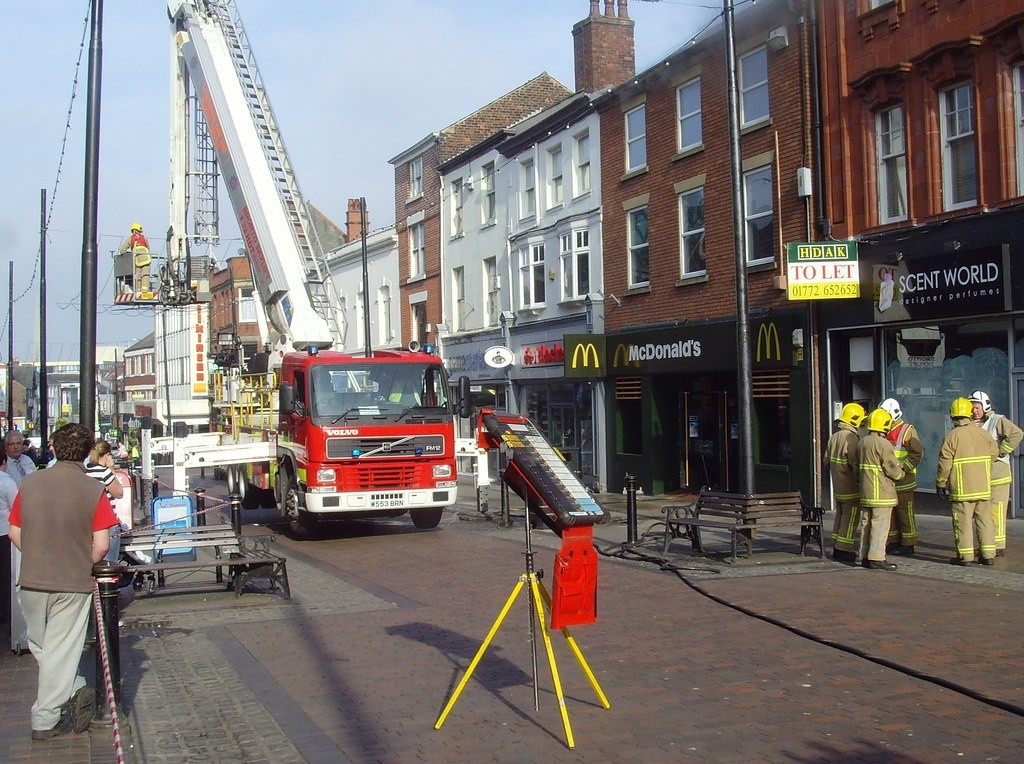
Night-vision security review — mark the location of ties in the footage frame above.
[15,461,25,478]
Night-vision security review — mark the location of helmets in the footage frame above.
[949,397,973,419]
[967,391,992,413]
[867,409,893,433]
[878,398,903,422]
[839,403,868,428]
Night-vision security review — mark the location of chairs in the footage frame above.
[315,371,334,413]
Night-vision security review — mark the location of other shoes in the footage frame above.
[977,557,993,565]
[833,549,861,562]
[886,543,914,555]
[950,557,971,566]
[975,548,1004,556]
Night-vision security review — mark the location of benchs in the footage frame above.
[662,485,827,561]
[118,516,292,602]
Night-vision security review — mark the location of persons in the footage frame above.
[0,430,123,626]
[120,223,151,292]
[968,391,1024,558]
[115,440,128,468]
[822,403,906,571]
[936,397,1000,566]
[877,398,923,556]
[8,424,119,740]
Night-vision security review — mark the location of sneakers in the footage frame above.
[70,688,96,733]
[32,716,70,741]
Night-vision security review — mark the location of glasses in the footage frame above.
[7,442,22,447]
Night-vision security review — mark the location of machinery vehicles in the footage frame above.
[113,0,472,540]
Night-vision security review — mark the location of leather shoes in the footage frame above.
[862,559,898,571]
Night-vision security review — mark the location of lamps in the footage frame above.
[943,240,961,252]
[766,25,789,51]
[882,250,905,264]
